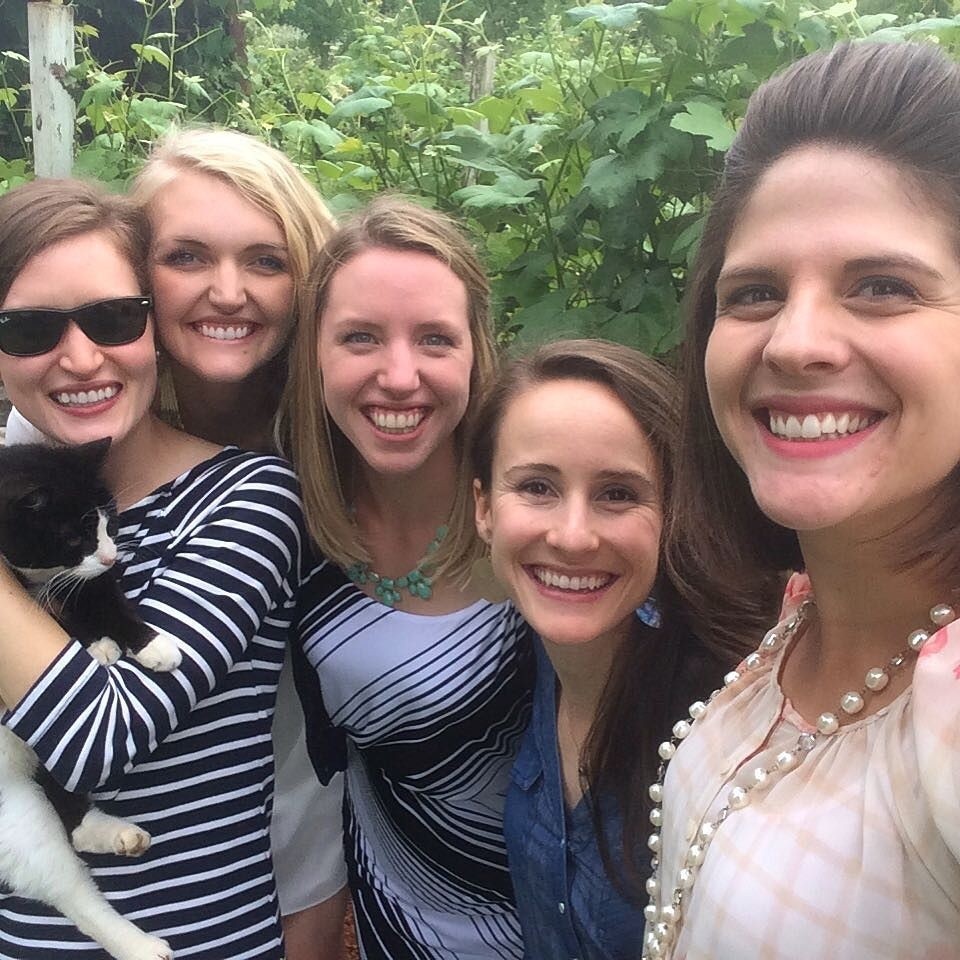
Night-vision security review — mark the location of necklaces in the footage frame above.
[562,701,581,758]
[644,597,956,960]
[344,465,461,606]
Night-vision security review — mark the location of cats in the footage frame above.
[0,436,179,960]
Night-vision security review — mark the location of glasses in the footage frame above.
[0,292,155,358]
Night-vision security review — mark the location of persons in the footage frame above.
[2,129,347,960]
[639,37,960,960]
[469,339,760,960]
[284,203,534,960]
[0,178,305,960]
